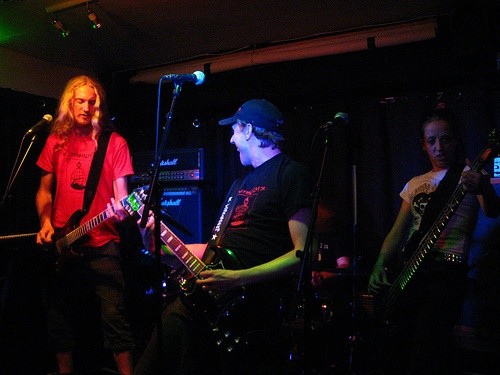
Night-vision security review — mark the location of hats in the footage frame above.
[218,98,285,133]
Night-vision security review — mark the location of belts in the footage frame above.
[427,252,465,265]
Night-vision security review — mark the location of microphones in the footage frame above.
[27,114,53,135]
[163,71,205,85]
[321,112,350,129]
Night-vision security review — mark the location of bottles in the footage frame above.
[311,243,336,295]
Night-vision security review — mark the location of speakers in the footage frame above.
[159,187,216,244]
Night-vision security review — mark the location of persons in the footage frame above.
[304,192,352,375]
[35,74,134,375]
[366,106,499,375]
[137,98,317,375]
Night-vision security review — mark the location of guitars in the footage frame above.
[350,125,500,332]
[122,191,245,348]
[30,183,153,270]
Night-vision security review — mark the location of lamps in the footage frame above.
[48,11,71,40]
[82,2,103,31]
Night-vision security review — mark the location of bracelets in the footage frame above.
[373,263,384,269]
